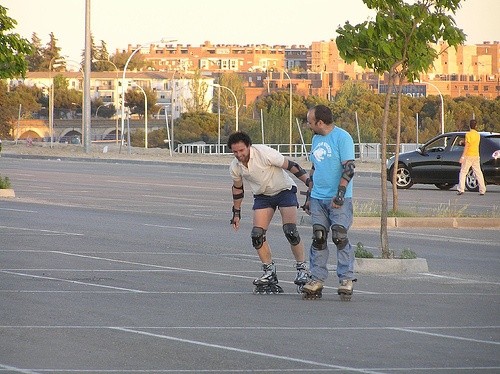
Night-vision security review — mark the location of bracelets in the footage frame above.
[460,156,464,159]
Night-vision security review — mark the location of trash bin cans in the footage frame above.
[71,136,80,144]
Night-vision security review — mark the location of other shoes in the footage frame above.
[479,193,485,196]
[456,191,463,195]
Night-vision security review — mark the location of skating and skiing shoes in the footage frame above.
[338,279,354,301]
[303,282,323,300]
[294,261,308,294]
[253,262,284,295]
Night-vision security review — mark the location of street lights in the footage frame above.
[121,39,176,146]
[212,84,239,132]
[126,82,148,149]
[91,59,120,145]
[49,55,68,149]
[270,66,293,158]
[413,80,445,135]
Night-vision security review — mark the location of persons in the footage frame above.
[302,104,358,302]
[456,120,487,196]
[228,132,313,295]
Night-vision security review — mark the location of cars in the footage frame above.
[387,129,500,192]
